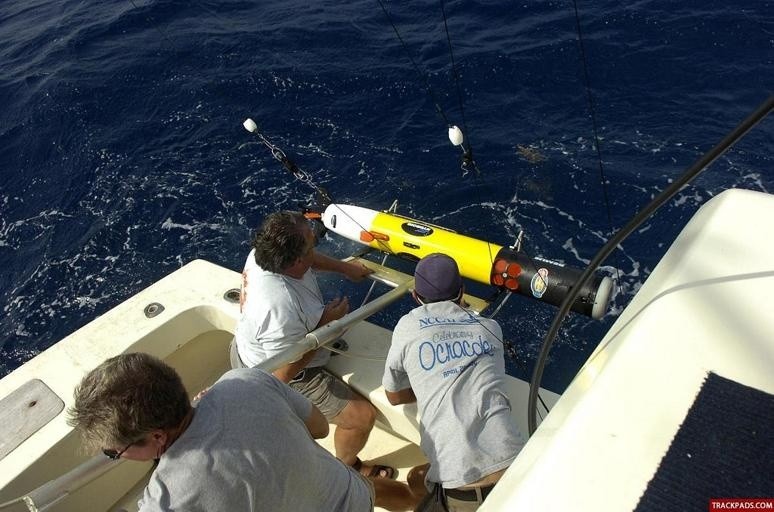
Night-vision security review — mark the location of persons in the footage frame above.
[381,252,527,512]
[228,210,395,481]
[64,351,423,512]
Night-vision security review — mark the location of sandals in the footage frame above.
[350,455,393,480]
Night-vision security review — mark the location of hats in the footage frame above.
[414,253,463,301]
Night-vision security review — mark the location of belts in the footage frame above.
[443,482,496,502]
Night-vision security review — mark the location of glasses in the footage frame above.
[101,438,146,461]
